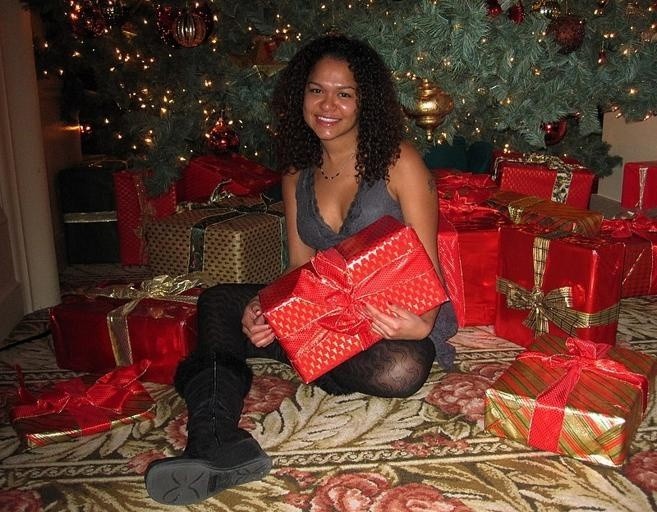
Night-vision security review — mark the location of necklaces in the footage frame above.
[317,151,355,185]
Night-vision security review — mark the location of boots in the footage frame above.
[245,334,348,397]
[144,350,272,506]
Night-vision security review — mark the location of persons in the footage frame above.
[142,30,458,505]
